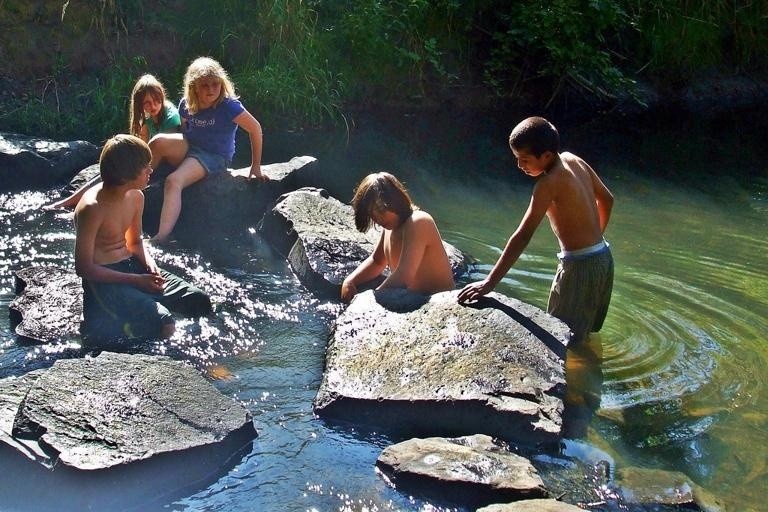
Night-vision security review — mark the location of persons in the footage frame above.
[75,134,258,381]
[341,172,454,299]
[147,56,269,238]
[456,118,614,363]
[43,74,182,211]
